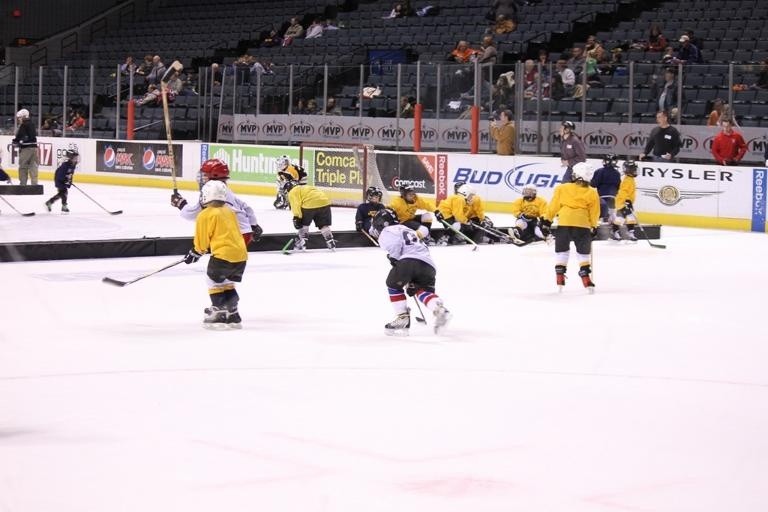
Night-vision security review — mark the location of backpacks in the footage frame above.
[627,42,648,52]
[415,3,440,17]
[616,67,634,76]
[149,92,162,106]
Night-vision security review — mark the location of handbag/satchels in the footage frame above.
[281,36,292,48]
[596,64,612,76]
[572,83,590,99]
[362,86,384,100]
[586,57,600,78]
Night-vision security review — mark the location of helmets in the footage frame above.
[570,161,595,182]
[15,108,30,118]
[199,179,228,205]
[561,119,576,130]
[277,164,301,182]
[274,154,290,170]
[372,207,400,234]
[601,152,619,169]
[199,159,231,180]
[522,184,537,198]
[400,183,414,197]
[65,149,79,159]
[366,186,385,201]
[623,160,638,177]
[454,182,467,196]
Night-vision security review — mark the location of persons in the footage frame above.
[373,207,449,335]
[2,0,767,250]
[185,179,248,330]
[540,162,601,295]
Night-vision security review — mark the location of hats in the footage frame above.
[610,47,624,53]
[679,34,689,43]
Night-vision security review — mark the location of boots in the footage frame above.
[323,234,337,249]
[554,264,566,287]
[625,227,639,241]
[46,199,53,212]
[293,239,308,251]
[203,306,242,324]
[430,301,449,331]
[609,229,623,240]
[61,202,70,212]
[384,311,411,329]
[578,269,597,288]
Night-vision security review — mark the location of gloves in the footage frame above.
[251,225,262,243]
[589,225,598,238]
[183,247,203,264]
[539,216,554,237]
[170,193,188,210]
[434,209,443,221]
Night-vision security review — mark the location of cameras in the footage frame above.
[488,116,494,121]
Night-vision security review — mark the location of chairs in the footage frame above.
[1,0,768,141]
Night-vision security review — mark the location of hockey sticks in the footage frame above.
[161,60,184,195]
[472,222,526,245]
[102,259,186,286]
[0,196,35,217]
[7,142,51,152]
[414,294,428,325]
[630,207,667,248]
[441,219,479,252]
[70,181,123,214]
